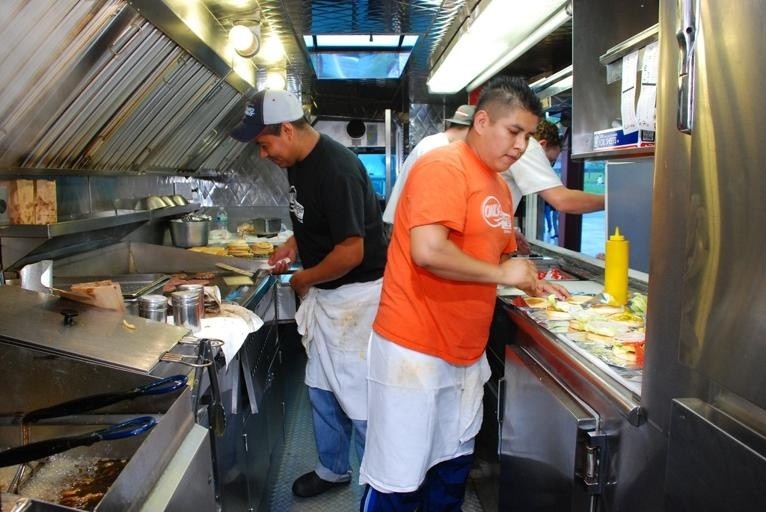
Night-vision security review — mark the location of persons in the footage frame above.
[230,89,392,499]
[358,76,570,511]
[498,117,606,255]
[382,106,474,224]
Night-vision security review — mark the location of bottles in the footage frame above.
[605,227,629,307]
[172,291,200,327]
[217,207,228,240]
[177,284,204,319]
[138,295,167,324]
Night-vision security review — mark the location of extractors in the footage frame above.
[0,0,324,178]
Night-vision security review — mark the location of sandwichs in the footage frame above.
[522,293,646,367]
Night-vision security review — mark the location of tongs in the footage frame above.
[191,339,226,437]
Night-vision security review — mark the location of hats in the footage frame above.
[228,86,304,142]
[444,104,477,126]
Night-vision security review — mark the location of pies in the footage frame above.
[188,240,274,259]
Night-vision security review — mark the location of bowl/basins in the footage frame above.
[169,217,209,247]
[252,217,282,236]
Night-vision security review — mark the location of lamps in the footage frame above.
[427,0,573,96]
[571,0,660,162]
[227,10,261,57]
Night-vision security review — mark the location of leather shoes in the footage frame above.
[291,471,350,499]
